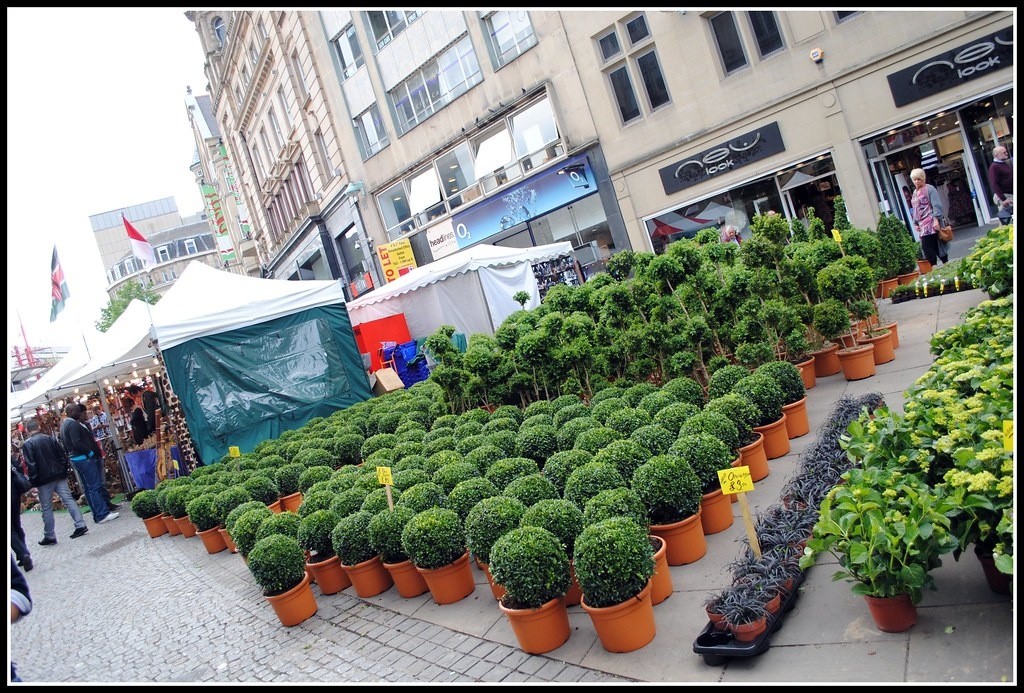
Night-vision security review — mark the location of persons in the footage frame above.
[726,225,743,245]
[122,398,152,446]
[768,211,777,215]
[988,146,1013,226]
[22,420,89,545]
[910,167,952,265]
[798,207,809,230]
[11,428,33,682]
[947,181,972,224]
[129,385,160,432]
[90,406,119,461]
[57,402,122,524]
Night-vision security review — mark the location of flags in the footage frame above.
[122,217,157,273]
[50,242,70,323]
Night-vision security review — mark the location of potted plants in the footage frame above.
[914,241,933,276]
[131,194,919,654]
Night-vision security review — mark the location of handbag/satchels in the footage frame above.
[11,465,33,494]
[997,206,1013,224]
[939,225,952,241]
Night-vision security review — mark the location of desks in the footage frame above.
[124,445,180,493]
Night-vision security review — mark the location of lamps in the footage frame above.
[345,180,366,202]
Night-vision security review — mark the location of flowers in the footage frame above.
[798,222,1013,606]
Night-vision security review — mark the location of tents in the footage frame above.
[695,201,733,222]
[345,241,585,351]
[10,259,375,489]
[647,210,719,235]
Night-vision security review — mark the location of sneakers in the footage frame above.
[99,512,119,523]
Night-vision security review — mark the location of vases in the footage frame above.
[860,592,917,634]
[974,544,1014,597]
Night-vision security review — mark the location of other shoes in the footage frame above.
[22,555,33,572]
[109,504,122,510]
[70,527,88,539]
[38,537,57,545]
